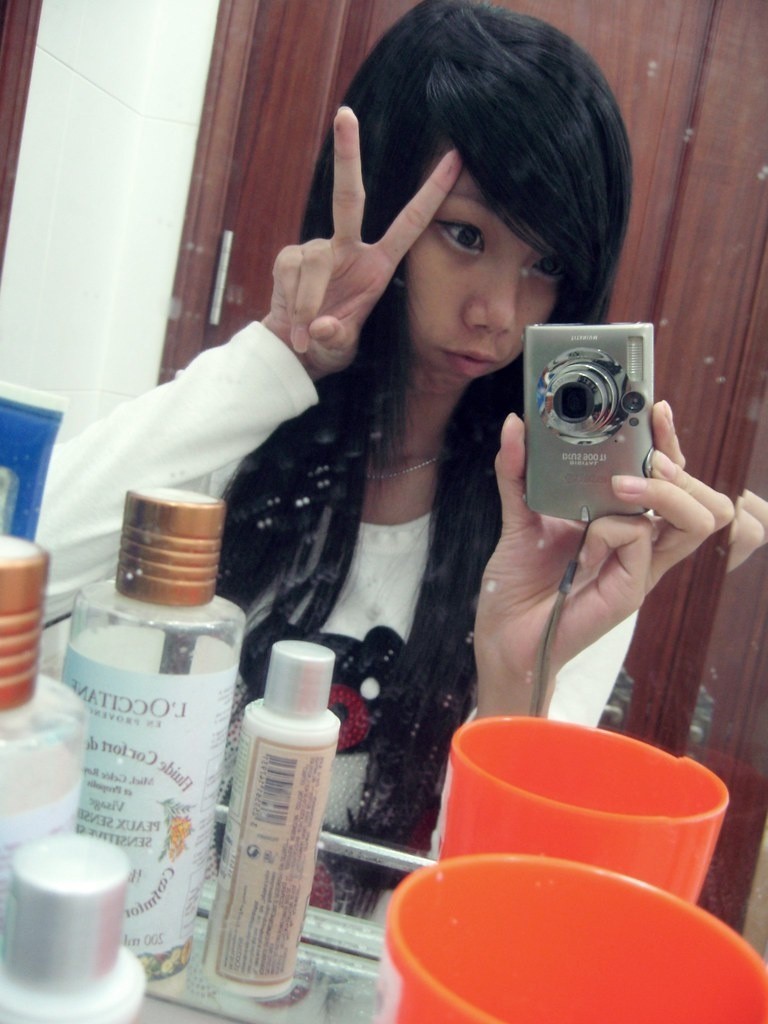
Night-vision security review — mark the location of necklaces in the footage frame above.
[366,456,440,480]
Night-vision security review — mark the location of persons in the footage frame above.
[727,489,768,574]
[26,0,736,925]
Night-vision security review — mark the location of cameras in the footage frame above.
[523,323,655,521]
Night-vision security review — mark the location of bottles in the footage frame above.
[0,533,90,947]
[202,634,344,1000]
[63,485,249,983]
[0,837,144,1024]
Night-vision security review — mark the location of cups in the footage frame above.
[437,715,732,909]
[385,856,767,1024]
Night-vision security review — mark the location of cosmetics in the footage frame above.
[207,638,339,1000]
[0,832,147,1024]
